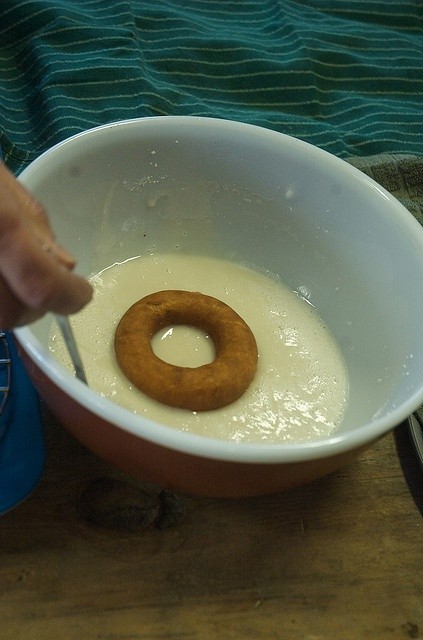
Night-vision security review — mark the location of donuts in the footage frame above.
[113,291,258,409]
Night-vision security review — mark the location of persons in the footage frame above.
[0,159,93,331]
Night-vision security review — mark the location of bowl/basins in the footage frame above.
[11,115,423,502]
